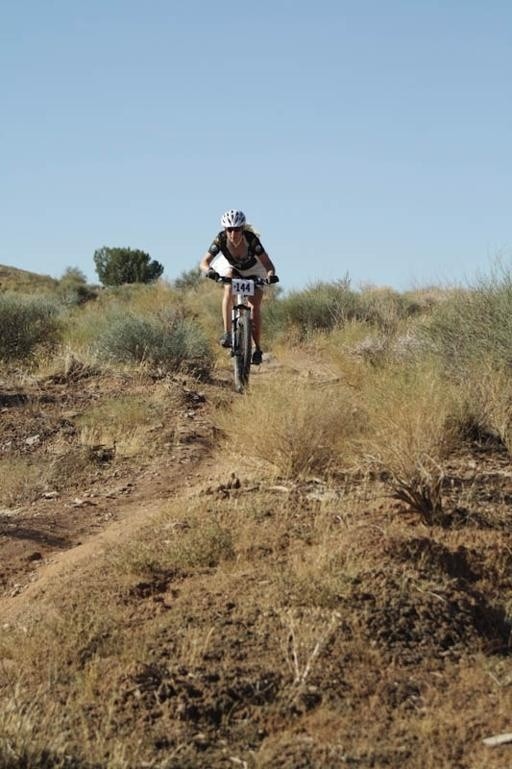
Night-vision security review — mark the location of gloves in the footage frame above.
[205,267,220,282]
[265,275,279,284]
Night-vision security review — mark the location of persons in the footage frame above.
[199,209,279,366]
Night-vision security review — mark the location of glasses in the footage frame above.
[226,227,241,233]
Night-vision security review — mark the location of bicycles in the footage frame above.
[206,274,269,391]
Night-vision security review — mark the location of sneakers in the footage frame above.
[220,332,233,347]
[252,348,263,365]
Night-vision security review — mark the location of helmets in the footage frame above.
[221,209,246,228]
